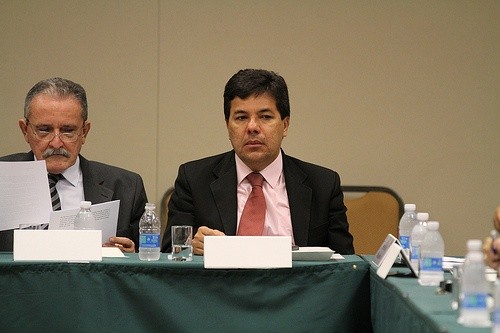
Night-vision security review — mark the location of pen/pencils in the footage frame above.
[110,243,130,247]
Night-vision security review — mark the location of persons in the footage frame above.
[482,206,500,281]
[0,77,148,254]
[160,68,356,255]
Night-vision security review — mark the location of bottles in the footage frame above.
[418,221,444,287]
[139,204,161,262]
[398,203,416,255]
[407,212,429,265]
[456,239,490,329]
[73,200,94,231]
[490,280,500,333]
[451,264,461,311]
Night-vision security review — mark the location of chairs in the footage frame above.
[342,185,405,254]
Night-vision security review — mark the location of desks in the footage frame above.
[0,237,369,333]
[359,250,500,333]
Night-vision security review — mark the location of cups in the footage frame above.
[170,226,192,263]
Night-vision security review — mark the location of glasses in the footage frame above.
[24,117,85,143]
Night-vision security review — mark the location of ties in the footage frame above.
[237,174,266,237]
[47,173,65,210]
